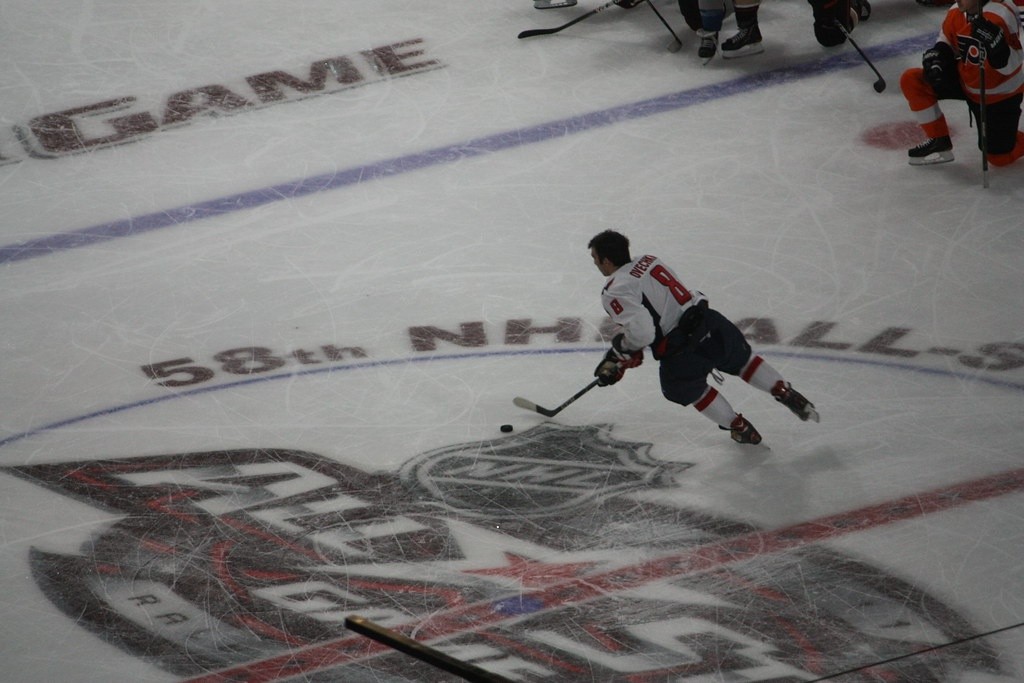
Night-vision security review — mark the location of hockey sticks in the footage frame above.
[517,1,621,39]
[500,424,515,435]
[513,361,624,418]
[645,1,683,54]
[975,0,991,189]
[824,1,887,94]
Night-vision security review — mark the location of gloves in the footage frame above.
[972,16,1010,69]
[595,333,643,387]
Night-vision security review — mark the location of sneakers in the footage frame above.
[908,135,954,165]
[728,412,762,445]
[696,28,718,65]
[775,382,819,422]
[923,48,949,86]
[722,24,765,59]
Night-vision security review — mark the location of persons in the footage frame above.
[589,231,820,452]
[533,0,871,64]
[900,1,1023,168]
